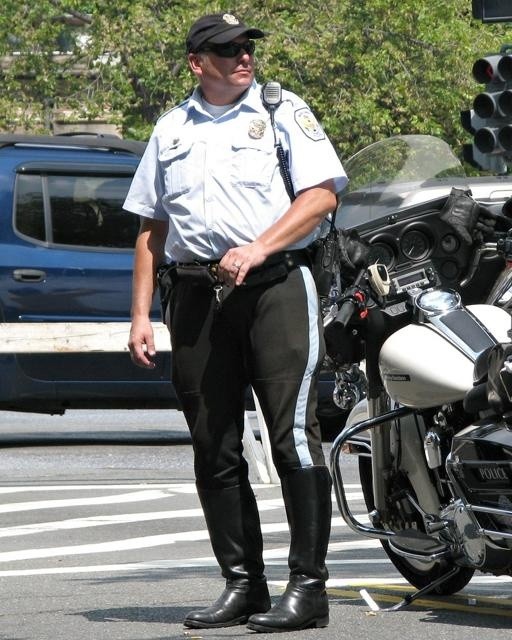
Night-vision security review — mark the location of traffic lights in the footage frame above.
[473,51,504,157]
[471,1,511,23]
[457,106,507,176]
[496,43,512,155]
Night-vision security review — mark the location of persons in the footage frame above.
[128,11,353,634]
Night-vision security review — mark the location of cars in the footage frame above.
[1,128,368,426]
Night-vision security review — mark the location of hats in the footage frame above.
[186,13,264,51]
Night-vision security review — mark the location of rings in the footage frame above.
[232,261,241,271]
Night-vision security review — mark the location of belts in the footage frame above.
[170,260,221,267]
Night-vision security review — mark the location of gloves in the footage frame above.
[440,187,498,244]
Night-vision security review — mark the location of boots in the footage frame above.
[246,465,333,633]
[183,480,271,628]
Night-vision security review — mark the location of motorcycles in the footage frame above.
[311,136,512,612]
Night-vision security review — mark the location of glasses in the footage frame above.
[202,41,256,57]
